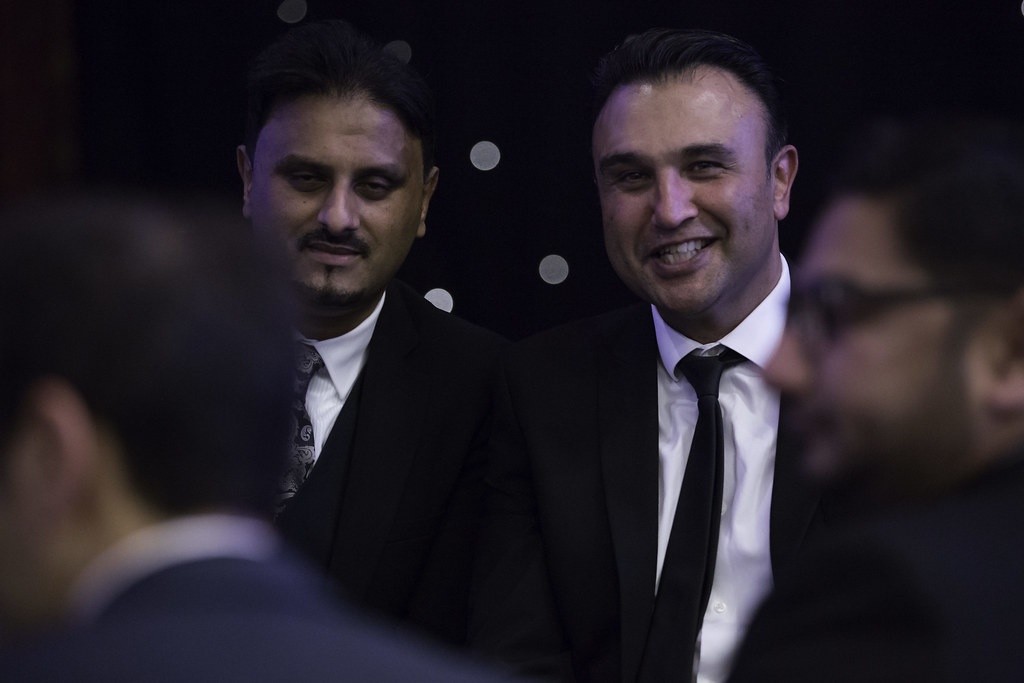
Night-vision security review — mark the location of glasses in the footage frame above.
[789,273,978,347]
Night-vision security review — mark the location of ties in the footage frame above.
[637,346,751,683]
[268,341,328,526]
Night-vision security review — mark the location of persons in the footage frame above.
[0,201,535,683]
[466,28,830,683]
[723,112,1024,683]
[237,21,511,683]
